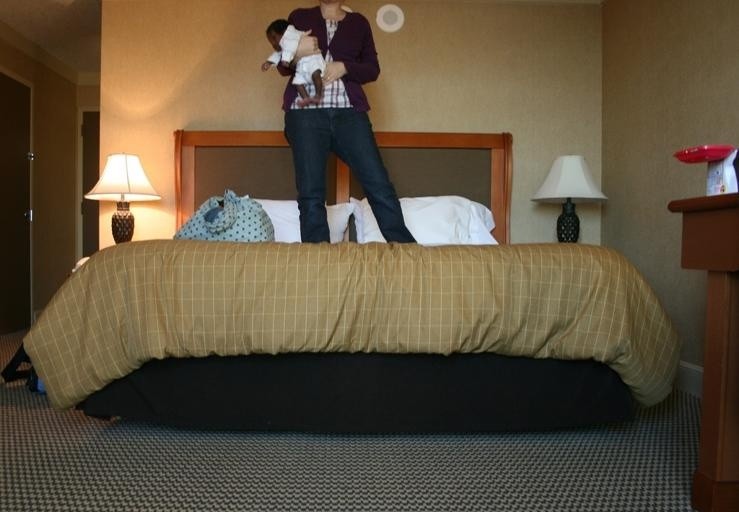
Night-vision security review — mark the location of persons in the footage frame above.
[276,0,416,243]
[261,19,322,105]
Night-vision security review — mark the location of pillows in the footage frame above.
[348,189,495,245]
[255,195,353,244]
[364,196,473,248]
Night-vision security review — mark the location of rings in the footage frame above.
[327,77,330,80]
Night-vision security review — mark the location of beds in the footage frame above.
[21,126,684,437]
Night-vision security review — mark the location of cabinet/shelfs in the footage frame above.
[664,188,739,511]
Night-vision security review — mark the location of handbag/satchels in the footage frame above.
[174,188,275,242]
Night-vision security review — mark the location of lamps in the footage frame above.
[527,153,608,245]
[84,153,162,245]
